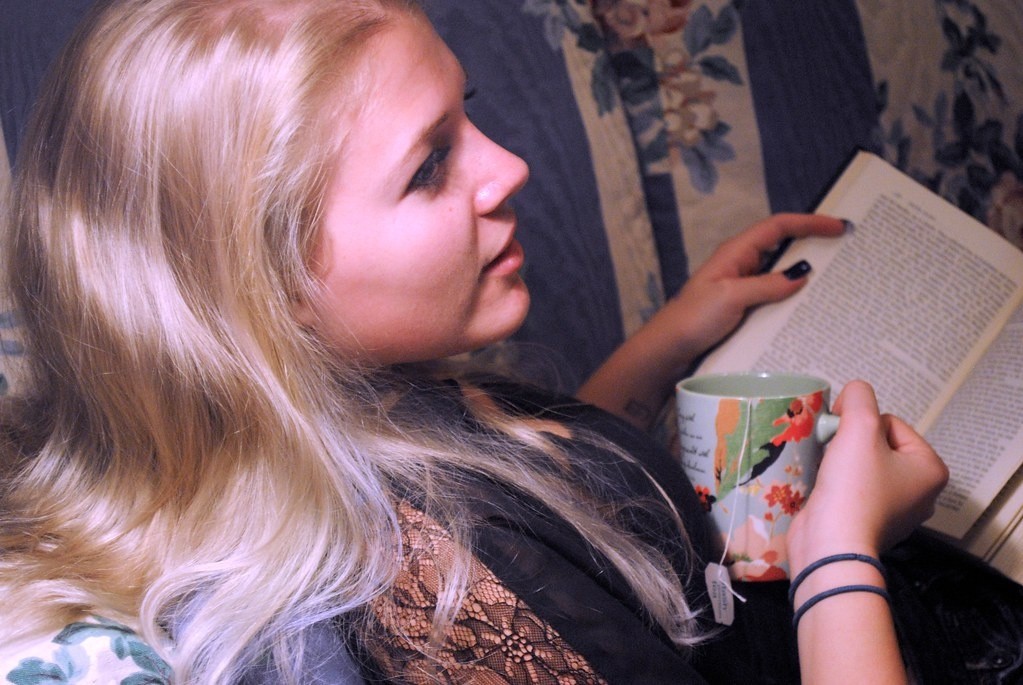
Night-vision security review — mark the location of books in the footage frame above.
[687,146,1022,595]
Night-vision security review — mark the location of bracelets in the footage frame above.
[788,549,896,632]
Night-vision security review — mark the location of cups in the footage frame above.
[675,372,841,584]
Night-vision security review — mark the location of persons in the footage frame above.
[1,2,1023,683]
